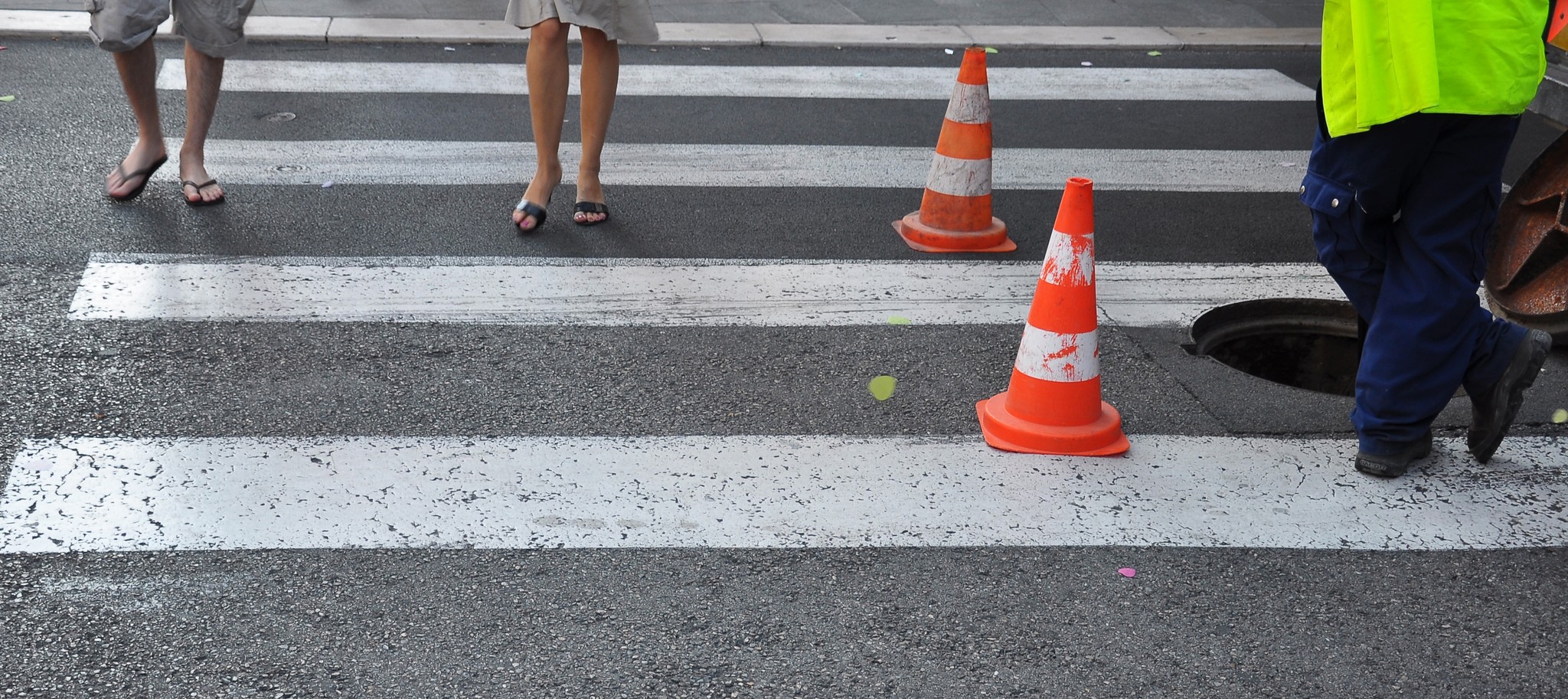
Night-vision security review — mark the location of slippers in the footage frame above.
[179,175,226,208]
[103,152,169,202]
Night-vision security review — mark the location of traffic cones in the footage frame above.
[976,176,1130,456]
[892,46,1017,252]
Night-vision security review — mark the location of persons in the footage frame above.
[1300,0,1551,479]
[504,0,660,233]
[87,0,256,207]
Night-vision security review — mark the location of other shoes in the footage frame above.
[1465,328,1553,464]
[1353,426,1434,476]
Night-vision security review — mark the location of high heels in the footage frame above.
[573,176,610,225]
[511,169,564,232]
